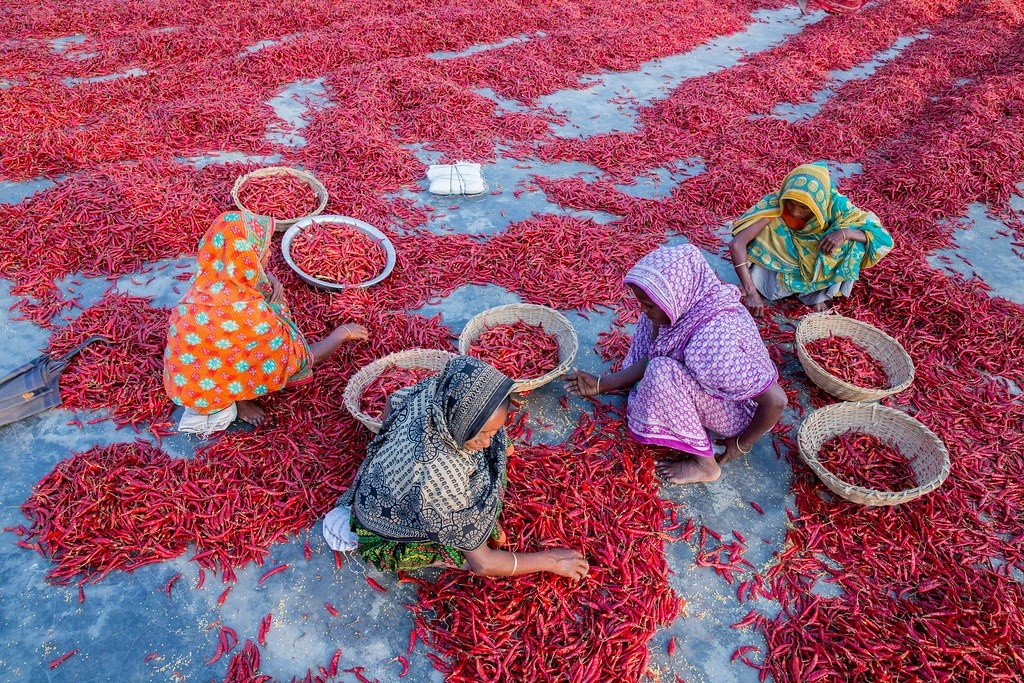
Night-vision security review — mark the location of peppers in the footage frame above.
[0,0,1024,683]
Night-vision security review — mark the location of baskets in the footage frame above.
[795,305,915,401]
[797,402,950,505]
[459,303,578,392]
[341,347,460,432]
[230,167,328,232]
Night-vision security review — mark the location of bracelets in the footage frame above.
[840,228,846,244]
[334,326,351,343]
[735,433,754,454]
[734,262,749,270]
[508,551,517,576]
[596,375,602,395]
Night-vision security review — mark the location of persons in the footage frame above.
[321,352,590,584]
[559,242,789,487]
[161,209,367,426]
[727,161,894,318]
